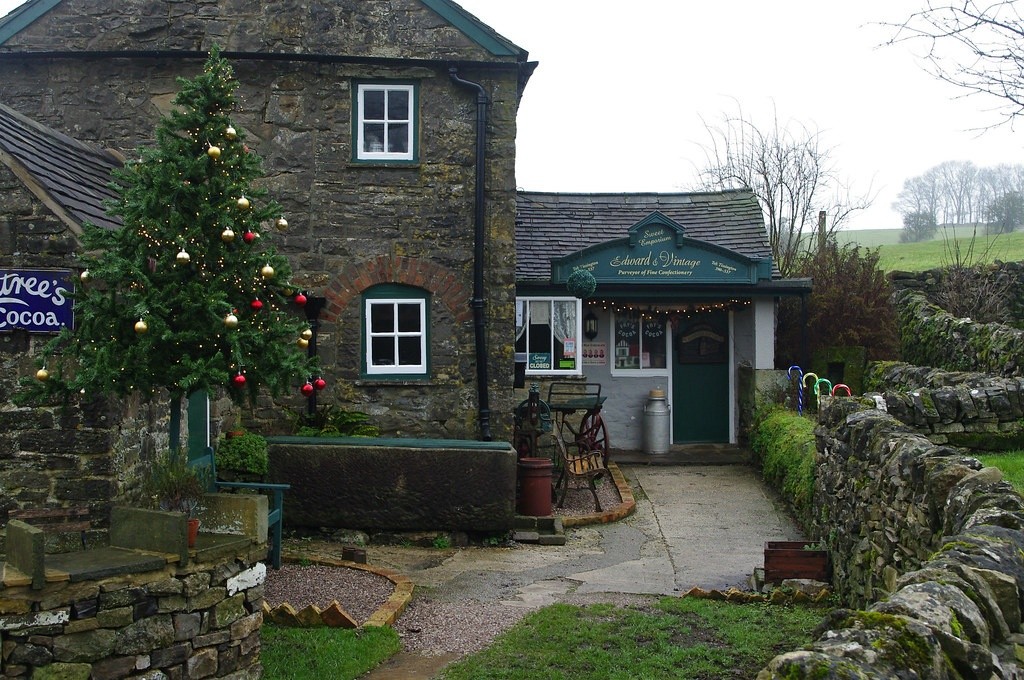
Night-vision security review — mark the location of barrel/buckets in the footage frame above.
[519,456,552,516]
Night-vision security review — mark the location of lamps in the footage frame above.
[583,312,598,342]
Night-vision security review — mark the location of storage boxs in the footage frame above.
[171,445,292,575]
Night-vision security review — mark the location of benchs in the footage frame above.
[550,419,605,512]
